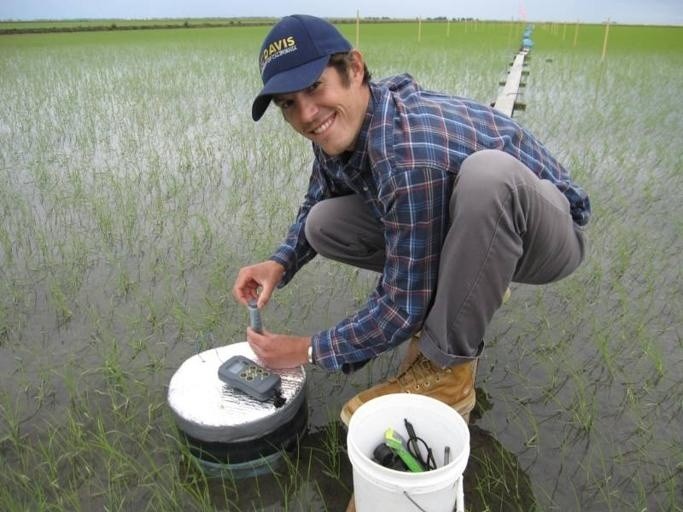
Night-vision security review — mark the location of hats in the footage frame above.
[252,14,354,122]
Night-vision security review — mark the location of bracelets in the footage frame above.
[307,339,314,366]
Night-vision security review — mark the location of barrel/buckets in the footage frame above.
[346,392,470,512]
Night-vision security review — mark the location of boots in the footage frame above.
[340,352,476,428]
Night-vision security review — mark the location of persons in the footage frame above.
[231,14,594,427]
